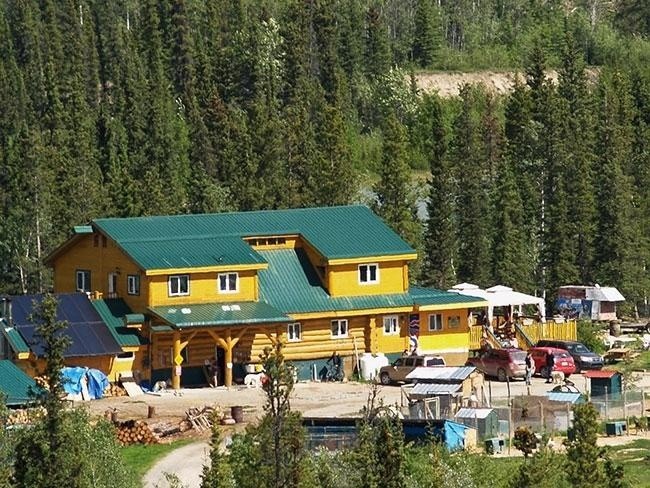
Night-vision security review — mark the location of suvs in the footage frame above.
[380,354,445,386]
[537,340,603,370]
[529,347,575,376]
[466,348,535,379]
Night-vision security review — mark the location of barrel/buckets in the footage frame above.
[231,406,246,422]
[609,319,620,336]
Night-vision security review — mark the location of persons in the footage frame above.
[208,357,218,389]
[402,349,417,356]
[484,338,494,349]
[327,351,343,377]
[509,335,518,348]
[525,352,532,385]
[545,350,555,383]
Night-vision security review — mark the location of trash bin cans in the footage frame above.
[606,421,627,437]
[231,406,244,422]
[485,438,505,455]
